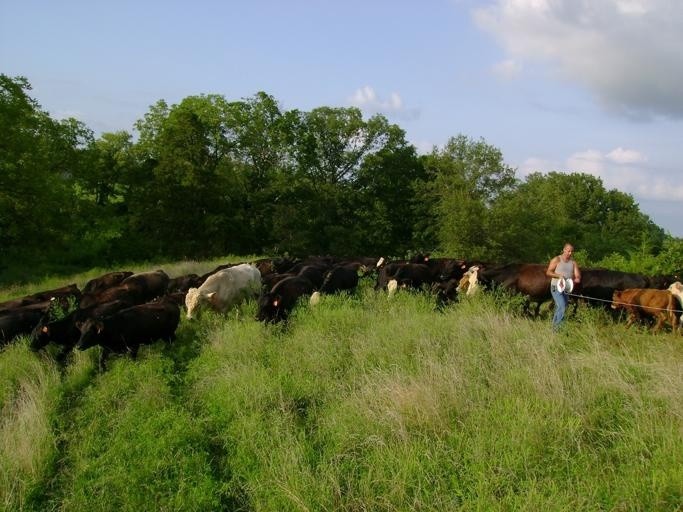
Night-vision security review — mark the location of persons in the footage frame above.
[544,242,581,337]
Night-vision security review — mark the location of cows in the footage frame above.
[375,258,682,337]
[0,256,385,375]
